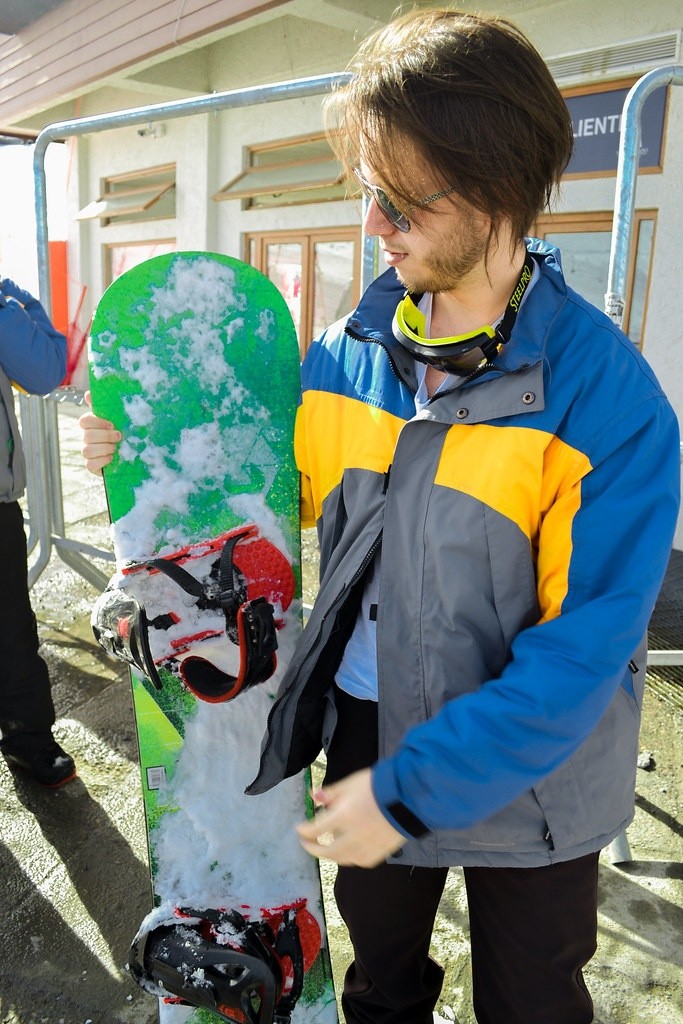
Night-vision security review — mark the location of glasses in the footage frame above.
[351,155,471,234]
[391,293,503,377]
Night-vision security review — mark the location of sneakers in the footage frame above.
[0,736,75,789]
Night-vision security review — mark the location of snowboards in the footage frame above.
[85,249,345,1024]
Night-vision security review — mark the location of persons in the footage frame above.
[0,275,77,788]
[79,9,681,1024]
